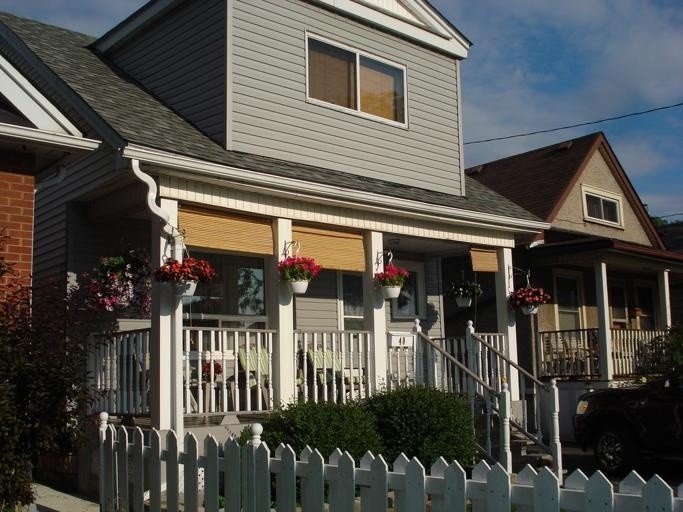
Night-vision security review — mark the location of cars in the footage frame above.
[570,362,682,476]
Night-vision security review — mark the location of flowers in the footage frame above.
[505,287,551,309]
[273,255,320,283]
[372,262,409,287]
[153,256,216,284]
[204,361,223,375]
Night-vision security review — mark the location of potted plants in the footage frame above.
[443,278,483,307]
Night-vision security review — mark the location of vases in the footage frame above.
[520,304,538,315]
[381,285,400,299]
[207,374,217,382]
[172,279,199,297]
[286,276,308,294]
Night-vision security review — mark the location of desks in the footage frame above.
[184,380,236,417]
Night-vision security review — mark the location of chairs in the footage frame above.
[238,345,305,411]
[301,348,366,404]
